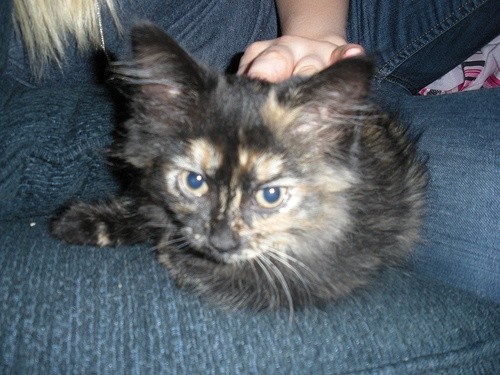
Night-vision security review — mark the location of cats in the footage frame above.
[47,19,434,323]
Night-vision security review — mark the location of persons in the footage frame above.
[0,1,499,306]
